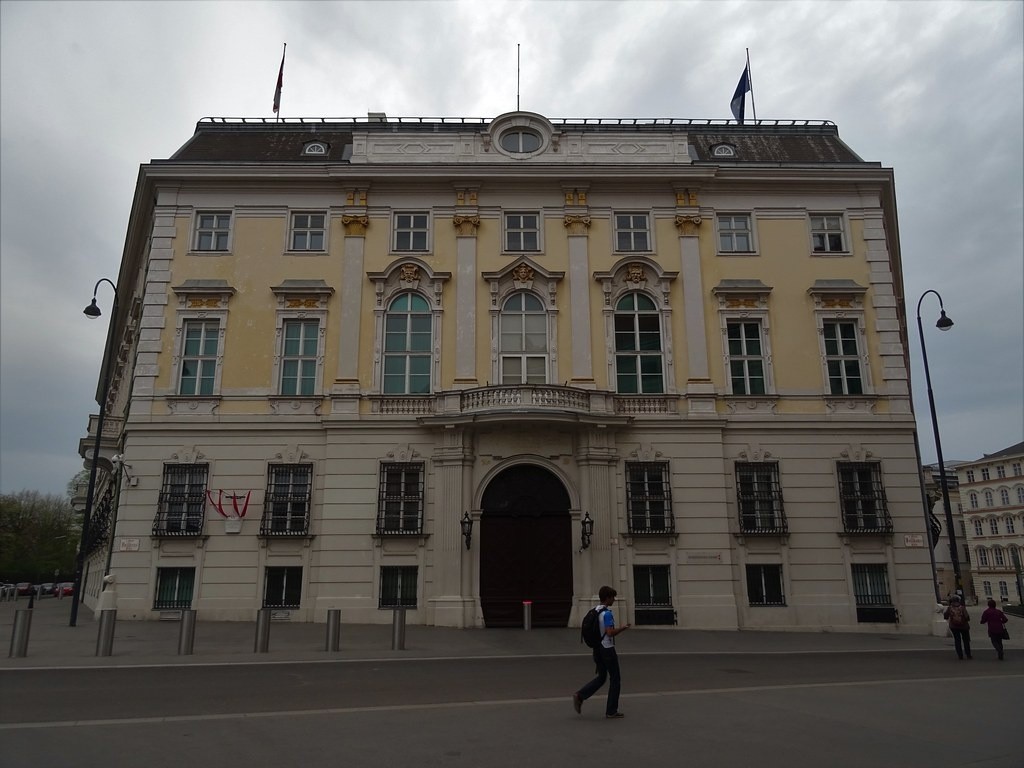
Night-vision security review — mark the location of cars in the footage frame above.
[42,583,55,595]
[15,583,35,596]
[55,582,74,596]
[0,582,16,596]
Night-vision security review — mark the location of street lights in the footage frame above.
[919,289,968,610]
[69,279,128,625]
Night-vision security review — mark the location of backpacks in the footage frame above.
[948,605,968,625]
[580,607,615,648]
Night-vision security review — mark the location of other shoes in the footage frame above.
[958,656,963,661]
[606,712,625,718]
[998,652,1004,660]
[572,692,584,714]
[967,655,974,660]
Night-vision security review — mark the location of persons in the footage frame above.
[943,595,974,660]
[573,586,632,719]
[980,599,1008,660]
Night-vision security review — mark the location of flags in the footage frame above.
[233,492,240,517]
[731,65,750,125]
[206,490,226,517]
[219,489,231,517]
[273,55,284,111]
[240,490,251,517]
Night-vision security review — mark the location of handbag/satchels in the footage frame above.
[1002,621,1010,640]
